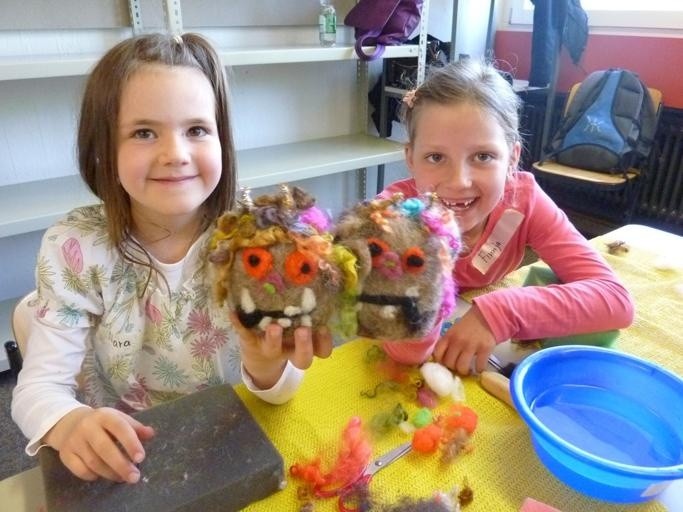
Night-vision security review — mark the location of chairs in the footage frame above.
[533,81,663,229]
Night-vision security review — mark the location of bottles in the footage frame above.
[318,1,337,44]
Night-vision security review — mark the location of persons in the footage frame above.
[9,31,331,487]
[379,54,636,378]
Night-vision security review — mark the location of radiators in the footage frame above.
[525,92,682,226]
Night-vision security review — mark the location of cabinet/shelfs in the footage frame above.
[0,0,428,371]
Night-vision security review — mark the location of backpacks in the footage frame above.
[546,68,658,175]
[344,0,423,61]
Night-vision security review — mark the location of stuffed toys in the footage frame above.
[334,190,466,345]
[201,184,356,345]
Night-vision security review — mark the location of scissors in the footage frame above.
[310,444,412,512]
[440,318,505,373]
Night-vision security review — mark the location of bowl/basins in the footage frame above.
[511,343,683,502]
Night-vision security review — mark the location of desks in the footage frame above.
[2,218,681,512]
